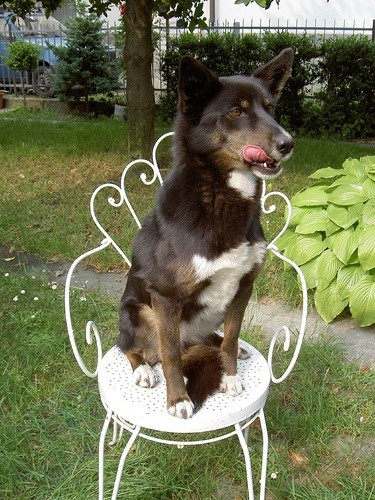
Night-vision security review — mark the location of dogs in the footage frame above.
[119,48,295,418]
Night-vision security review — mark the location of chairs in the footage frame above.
[64,130,307,500]
